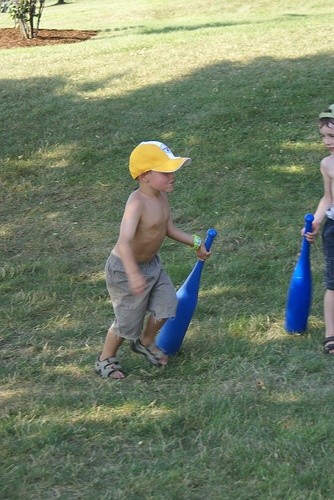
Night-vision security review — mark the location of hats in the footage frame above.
[319,104,334,118]
[129,139,191,180]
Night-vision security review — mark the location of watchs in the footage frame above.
[191,235,201,250]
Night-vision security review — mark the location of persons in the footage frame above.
[301,104,334,355]
[94,140,211,380]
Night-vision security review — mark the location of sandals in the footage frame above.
[94,355,126,379]
[129,337,167,368]
[322,336,334,353]
